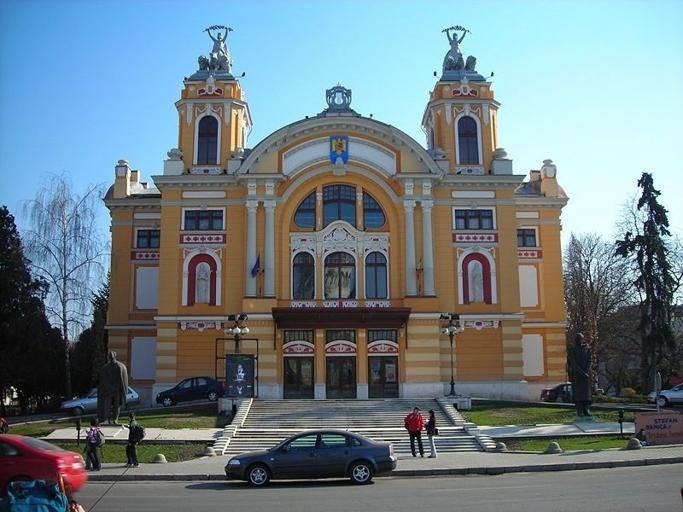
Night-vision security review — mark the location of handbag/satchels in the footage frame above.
[435,429,439,435]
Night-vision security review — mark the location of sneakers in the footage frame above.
[122,463,141,468]
[413,453,433,458]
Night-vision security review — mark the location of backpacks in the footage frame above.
[93,425,105,448]
[134,423,146,445]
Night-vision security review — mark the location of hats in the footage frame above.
[414,407,421,411]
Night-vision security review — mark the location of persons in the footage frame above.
[403,407,424,457]
[86,415,101,471]
[122,411,139,467]
[445,28,466,58]
[0,412,9,434]
[235,363,245,395]
[89,350,128,424]
[423,409,437,458]
[566,331,593,416]
[206,27,228,57]
[82,436,97,471]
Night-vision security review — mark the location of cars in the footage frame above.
[647,383,683,411]
[60,384,142,417]
[0,432,88,503]
[224,428,398,486]
[541,383,604,405]
[154,375,225,408]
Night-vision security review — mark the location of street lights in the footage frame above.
[224,312,251,352]
[439,312,465,396]
